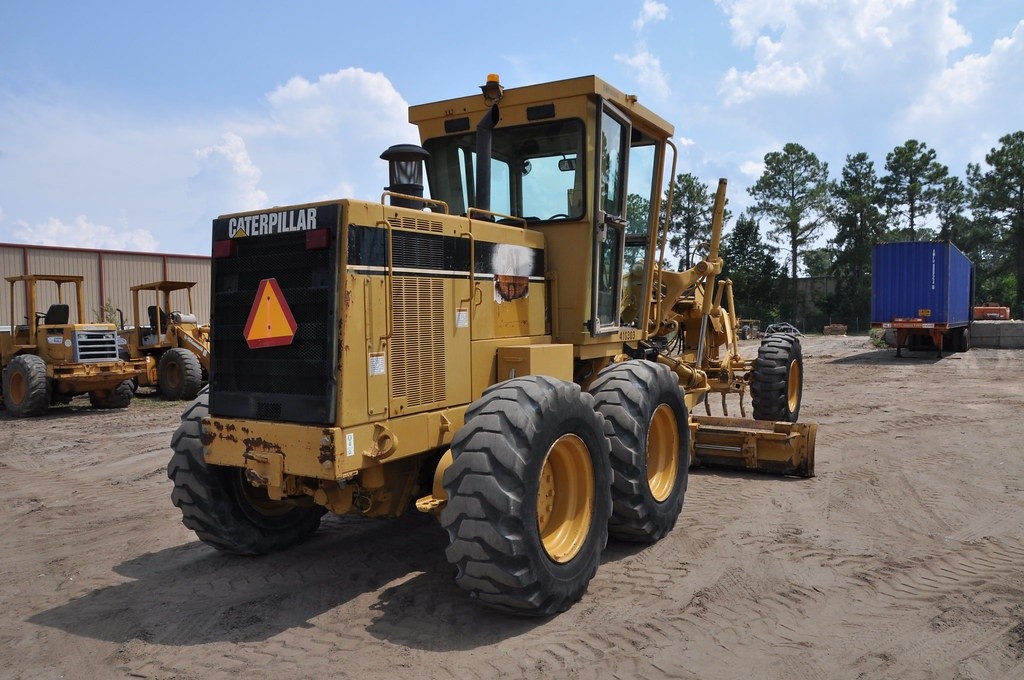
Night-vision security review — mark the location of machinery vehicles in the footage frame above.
[0,273,149,418]
[116,280,210,402]
[168,73,818,619]
[735,319,760,340]
